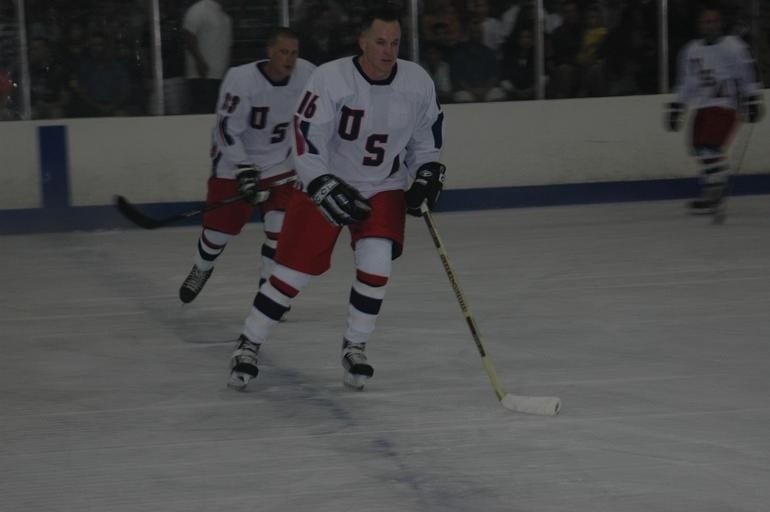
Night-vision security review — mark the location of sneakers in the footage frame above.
[177,264,217,304]
[228,329,263,378]
[337,336,374,377]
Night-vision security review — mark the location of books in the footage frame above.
[668,1,770,92]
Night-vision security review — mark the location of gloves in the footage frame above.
[660,98,688,133]
[403,160,446,215]
[742,87,764,125]
[306,171,375,226]
[235,165,273,206]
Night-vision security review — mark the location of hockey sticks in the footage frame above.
[113,175,297,229]
[420,199,561,417]
[713,123,755,224]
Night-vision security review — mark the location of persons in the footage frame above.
[226,10,447,382]
[182,1,233,115]
[418,1,663,100]
[10,2,150,121]
[663,4,766,221]
[303,16,350,66]
[174,26,342,316]
